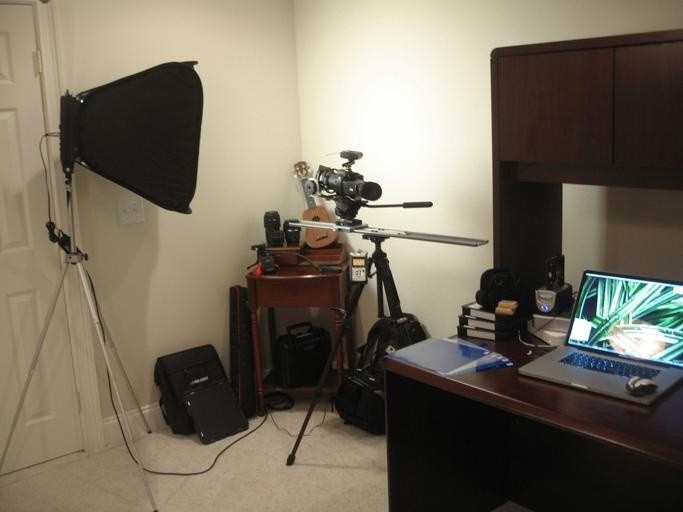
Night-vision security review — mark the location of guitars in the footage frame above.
[295,161,339,249]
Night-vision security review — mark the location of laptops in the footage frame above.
[518,270,683,408]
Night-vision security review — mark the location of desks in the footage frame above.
[384,336,683,512]
[246,256,350,417]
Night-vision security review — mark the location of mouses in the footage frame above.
[627,375,658,396]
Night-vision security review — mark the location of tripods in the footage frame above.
[285,248,399,465]
[0,251,156,512]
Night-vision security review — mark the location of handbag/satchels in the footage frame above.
[155,344,229,434]
[335,370,386,434]
[354,314,427,370]
[274,322,331,388]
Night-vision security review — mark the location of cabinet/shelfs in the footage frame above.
[490,28,683,190]
